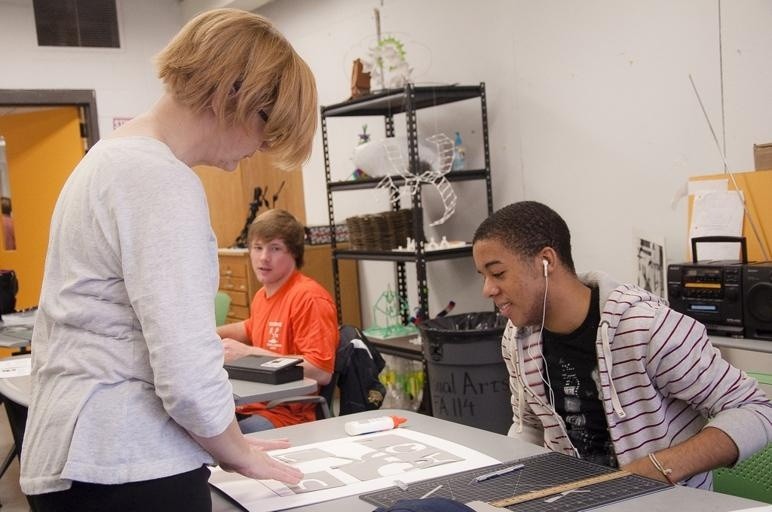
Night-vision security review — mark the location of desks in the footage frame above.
[210,409,772,512]
[0,352,316,454]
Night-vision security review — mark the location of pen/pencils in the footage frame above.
[476,463,524,481]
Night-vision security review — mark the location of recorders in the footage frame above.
[665,236,772,342]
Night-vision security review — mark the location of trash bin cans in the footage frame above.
[419,311,514,436]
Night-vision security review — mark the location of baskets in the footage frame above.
[345,206,426,253]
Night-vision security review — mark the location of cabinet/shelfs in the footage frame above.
[215,242,363,332]
[319,81,495,415]
[710,334,771,402]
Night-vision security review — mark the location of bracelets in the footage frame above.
[642,451,686,488]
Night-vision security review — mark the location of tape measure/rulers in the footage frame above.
[488,470,632,507]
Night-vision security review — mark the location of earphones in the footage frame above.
[528,262,555,415]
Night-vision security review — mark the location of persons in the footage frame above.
[214,206,341,436]
[17,6,317,512]
[469,199,768,487]
